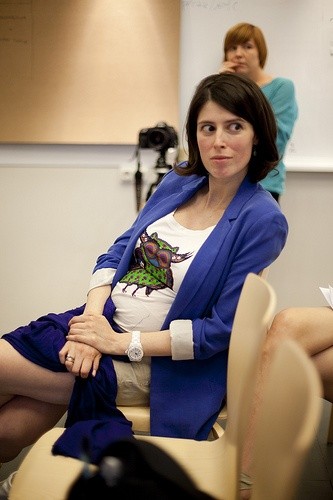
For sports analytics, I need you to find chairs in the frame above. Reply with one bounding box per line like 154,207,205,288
245,335,324,500
97,267,265,440
7,272,276,500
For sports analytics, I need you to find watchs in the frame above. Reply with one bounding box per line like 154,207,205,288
124,330,144,364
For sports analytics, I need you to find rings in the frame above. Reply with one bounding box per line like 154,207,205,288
65,355,74,363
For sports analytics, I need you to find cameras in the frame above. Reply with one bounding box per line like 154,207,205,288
139,125,179,150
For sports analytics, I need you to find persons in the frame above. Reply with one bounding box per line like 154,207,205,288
220,22,299,208
265,304,333,406
0,69,290,466
